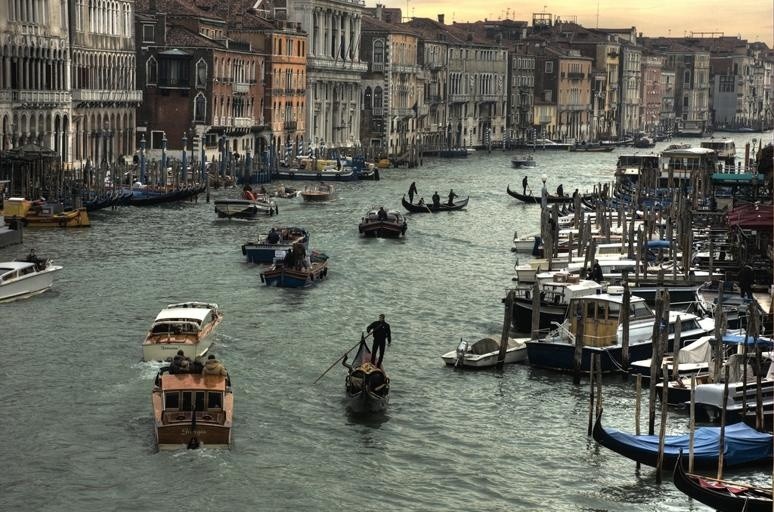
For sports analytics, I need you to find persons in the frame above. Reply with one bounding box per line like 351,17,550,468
366,314,391,368
557,184,563,197
417,198,425,206
26,249,41,269
202,355,227,375
283,242,312,274
572,189,581,198
267,228,278,244
432,191,440,207
368,205,386,220
408,182,417,204
168,350,203,374
336,156,341,170
522,176,527,196
447,189,458,206
587,259,603,282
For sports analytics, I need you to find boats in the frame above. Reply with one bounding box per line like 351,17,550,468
343,332,390,415
358,205,408,239
0,251,63,307
141,298,222,361
442,334,532,370
444,148,477,156
257,241,332,291
401,191,472,217
149,363,234,452
243,225,308,265
